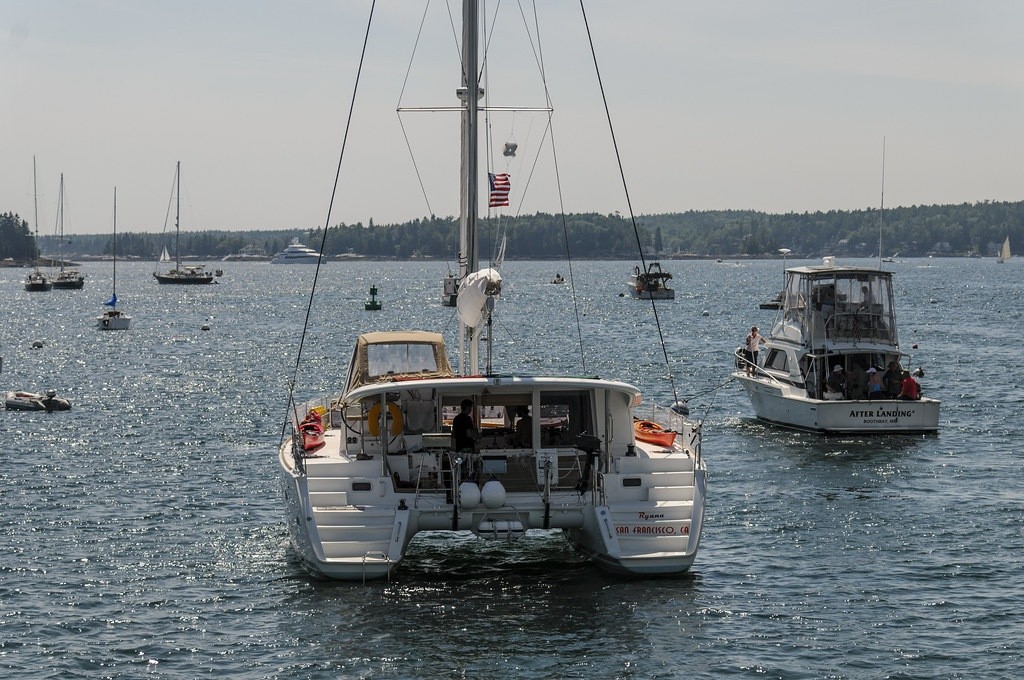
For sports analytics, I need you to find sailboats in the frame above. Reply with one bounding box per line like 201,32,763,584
996,236,1011,265
24,154,53,291
275,0,708,583
49,173,84,289
97,186,131,330
153,161,213,283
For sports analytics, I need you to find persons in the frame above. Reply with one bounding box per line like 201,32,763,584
860,286,876,311
514,408,532,448
882,361,901,399
746,327,766,376
897,371,917,400
827,359,865,400
451,398,483,480
866,367,882,400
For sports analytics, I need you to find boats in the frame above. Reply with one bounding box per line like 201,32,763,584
270,236,325,264
731,136,941,435
716,259,721,262
760,288,787,309
4,392,72,412
882,256,894,262
627,258,675,299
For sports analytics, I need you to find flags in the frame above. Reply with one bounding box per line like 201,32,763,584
488,173,511,207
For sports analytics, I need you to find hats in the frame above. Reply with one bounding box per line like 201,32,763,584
887,361,897,368
866,368,877,373
833,365,843,372
